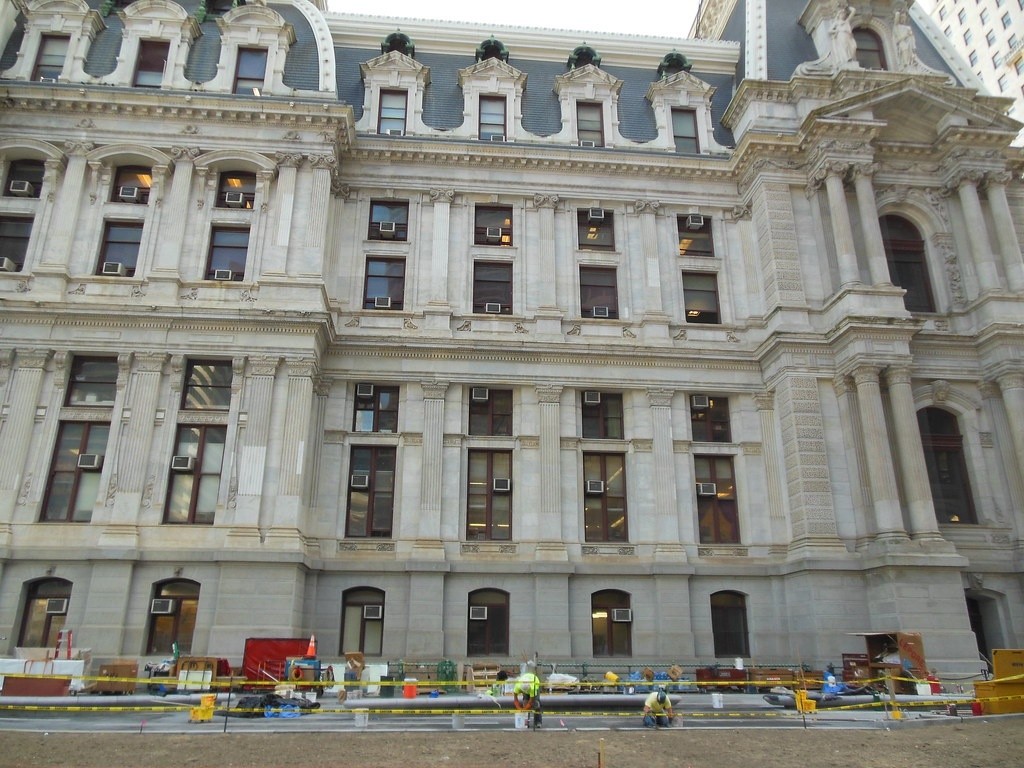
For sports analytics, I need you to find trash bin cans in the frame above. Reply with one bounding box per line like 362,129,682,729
404,679,417,698
380,676,394,697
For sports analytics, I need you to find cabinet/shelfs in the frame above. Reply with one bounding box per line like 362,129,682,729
97,663,139,695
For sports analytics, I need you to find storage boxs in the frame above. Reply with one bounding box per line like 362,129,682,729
973,649,1024,713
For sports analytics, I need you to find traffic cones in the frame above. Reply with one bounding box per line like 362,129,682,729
304,633,318,659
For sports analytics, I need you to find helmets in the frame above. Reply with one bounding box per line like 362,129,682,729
657,691,666,703
521,680,529,692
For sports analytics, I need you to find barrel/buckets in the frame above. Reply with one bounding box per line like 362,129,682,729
403,678,417,698
306,692,317,703
734,658,743,669
514,712,529,729
354,713,369,727
452,715,464,729
604,671,619,681
712,693,723,709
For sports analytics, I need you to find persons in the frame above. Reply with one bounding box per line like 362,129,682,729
512,673,542,728
822,664,848,693
345,657,364,690
643,688,673,728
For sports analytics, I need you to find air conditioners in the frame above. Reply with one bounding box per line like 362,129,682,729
486,227,502,242
692,394,710,409
351,384,374,488
697,480,717,497
40,76,57,83
585,479,605,495
584,391,601,404
103,261,127,275
362,606,381,620
608,607,633,621
485,303,501,313
593,305,609,318
467,606,489,622
170,453,195,472
148,598,176,614
47,598,70,614
493,478,510,491
375,222,395,309
118,186,142,202
474,386,488,402
78,453,100,470
215,270,234,280
588,209,604,222
0,255,17,272
9,180,34,196
686,215,703,229
224,191,245,206
387,128,594,149
